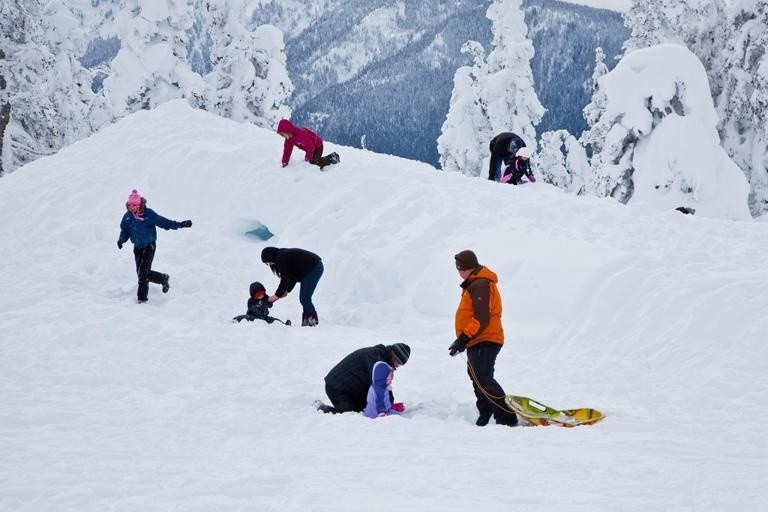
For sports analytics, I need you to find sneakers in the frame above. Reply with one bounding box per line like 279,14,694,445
160,274,170,293
476,412,519,427
329,152,340,164
312,398,323,411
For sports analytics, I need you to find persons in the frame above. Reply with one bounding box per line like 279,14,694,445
500,147,536,185
246,281,292,325
487,132,527,183
314,341,410,414
260,246,324,326
446,249,518,426
363,361,402,418
277,118,340,171
116,189,192,304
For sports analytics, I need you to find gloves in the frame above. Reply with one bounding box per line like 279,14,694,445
518,174,537,184
117,240,126,250
179,219,192,228
447,333,473,358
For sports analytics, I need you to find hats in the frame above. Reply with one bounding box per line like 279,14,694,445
453,249,481,272
506,138,524,153
389,342,410,366
515,147,530,159
126,193,142,206
